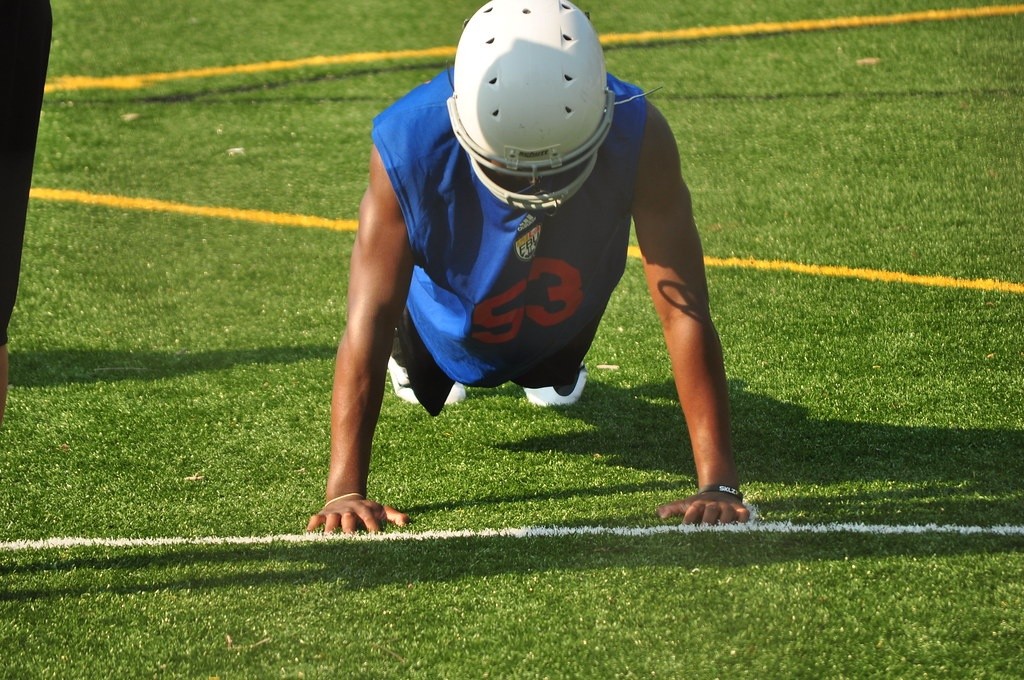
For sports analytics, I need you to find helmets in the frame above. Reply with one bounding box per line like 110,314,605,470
447,0,615,211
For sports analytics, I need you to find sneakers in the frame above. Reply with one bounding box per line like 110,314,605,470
388,355,465,406
523,362,587,408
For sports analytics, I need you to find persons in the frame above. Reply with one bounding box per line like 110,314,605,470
0,0,54,439
302,0,751,533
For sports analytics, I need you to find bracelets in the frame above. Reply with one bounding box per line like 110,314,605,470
696,485,744,502
325,493,365,507
